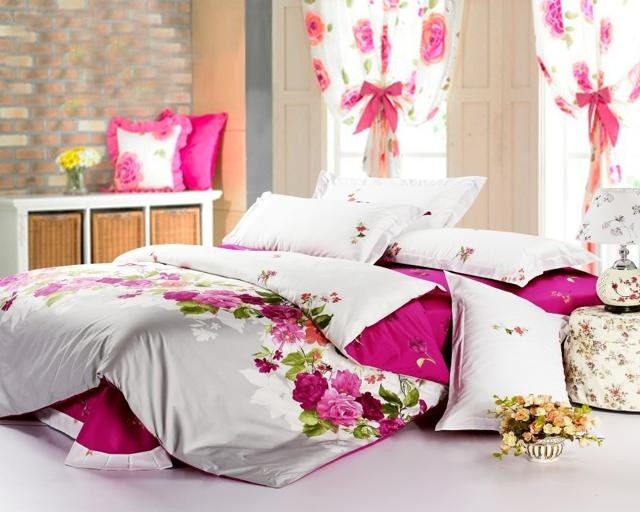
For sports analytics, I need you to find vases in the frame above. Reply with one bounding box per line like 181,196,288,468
61,164,88,197
519,437,567,463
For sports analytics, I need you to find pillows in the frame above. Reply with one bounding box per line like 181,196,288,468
431,267,577,436
308,171,487,232
98,115,191,193
226,189,421,266
386,225,603,287
159,107,229,191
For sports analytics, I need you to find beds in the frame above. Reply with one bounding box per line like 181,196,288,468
2,172,605,491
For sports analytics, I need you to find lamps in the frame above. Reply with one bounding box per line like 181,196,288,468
572,188,639,313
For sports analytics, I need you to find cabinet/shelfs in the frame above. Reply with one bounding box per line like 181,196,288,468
1,190,223,279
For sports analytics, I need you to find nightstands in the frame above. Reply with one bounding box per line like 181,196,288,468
565,304,640,417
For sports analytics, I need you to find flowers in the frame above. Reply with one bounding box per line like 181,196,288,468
57,146,99,190
481,390,604,459
